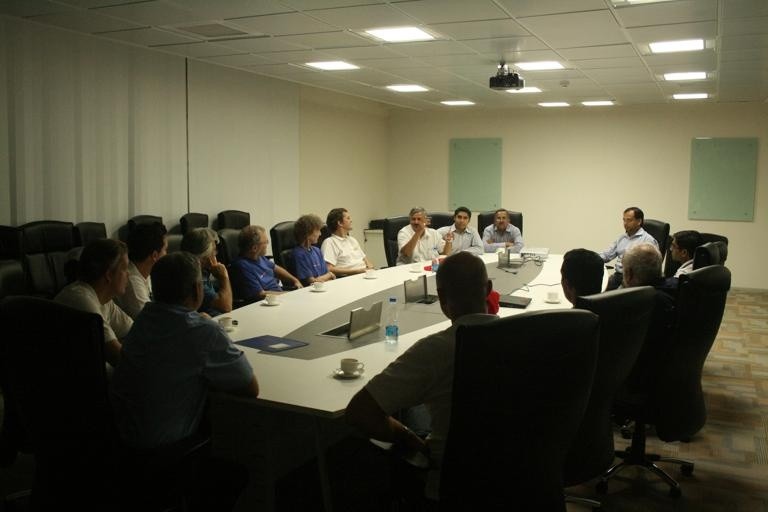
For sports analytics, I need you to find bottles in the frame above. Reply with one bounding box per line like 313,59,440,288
231,320,240,339
614,257,623,273
383,297,402,349
431,247,439,272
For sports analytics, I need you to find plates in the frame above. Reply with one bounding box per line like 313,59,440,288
308,287,326,292
408,269,424,273
331,366,366,380
362,274,377,279
215,327,235,333
260,301,282,306
543,299,562,303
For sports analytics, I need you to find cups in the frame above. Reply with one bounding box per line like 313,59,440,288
365,269,375,275
495,247,505,255
265,294,280,305
217,316,233,329
545,292,558,301
340,357,364,374
409,261,422,270
310,282,325,289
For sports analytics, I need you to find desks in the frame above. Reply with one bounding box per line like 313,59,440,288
211,249,614,512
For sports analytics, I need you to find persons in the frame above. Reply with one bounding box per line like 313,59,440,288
51,225,258,512
396,206,523,266
345,253,503,498
231,209,375,311
559,206,698,440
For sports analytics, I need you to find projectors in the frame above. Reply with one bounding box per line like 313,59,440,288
519,246,549,261
489,73,525,90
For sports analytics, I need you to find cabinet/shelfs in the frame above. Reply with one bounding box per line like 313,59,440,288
359,229,390,271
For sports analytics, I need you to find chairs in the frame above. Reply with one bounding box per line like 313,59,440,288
0,297,264,512
382,209,524,266
382,308,602,512
614,214,731,278
594,265,731,498
555,286,656,512
0,211,330,311
